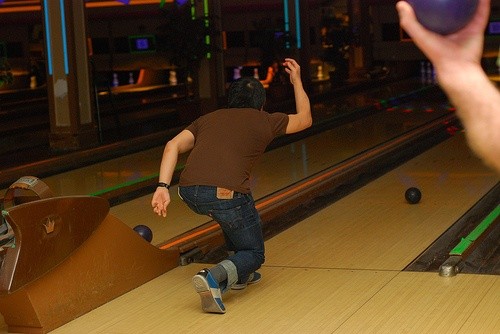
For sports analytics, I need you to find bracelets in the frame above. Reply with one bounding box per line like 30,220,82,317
156,182,171,189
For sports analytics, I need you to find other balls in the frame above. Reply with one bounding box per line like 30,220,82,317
405,188,421,203
133,225,152,242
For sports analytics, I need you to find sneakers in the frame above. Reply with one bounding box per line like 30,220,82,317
192,267,226,313
230,271,262,289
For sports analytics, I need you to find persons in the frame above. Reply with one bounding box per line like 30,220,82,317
153,59,312,313
398,0,500,176
